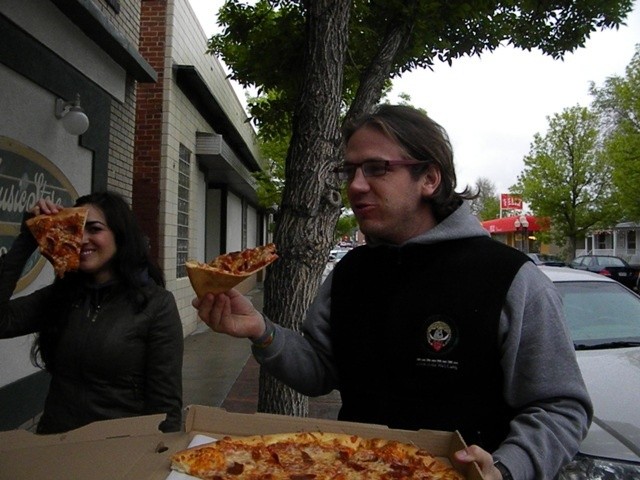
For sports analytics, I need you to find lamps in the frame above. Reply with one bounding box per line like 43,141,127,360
54,91,89,136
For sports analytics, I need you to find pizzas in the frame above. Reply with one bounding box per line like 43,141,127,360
171,433,462,476
185,243,280,298
26,205,91,279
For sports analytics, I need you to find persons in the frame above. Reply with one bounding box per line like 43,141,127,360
0,189,183,436
192,105,593,480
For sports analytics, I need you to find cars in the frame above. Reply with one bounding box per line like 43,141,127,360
535,264,639,480
331,251,347,267
525,253,564,266
568,255,638,289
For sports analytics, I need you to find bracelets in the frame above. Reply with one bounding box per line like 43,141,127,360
491,456,514,480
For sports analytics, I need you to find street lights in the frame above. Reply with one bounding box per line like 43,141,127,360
514,215,529,253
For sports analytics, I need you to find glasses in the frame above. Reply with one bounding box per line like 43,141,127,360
333,160,431,178
